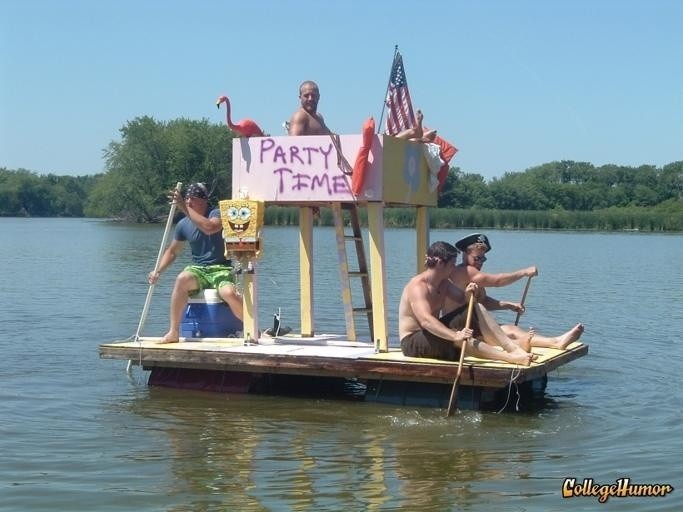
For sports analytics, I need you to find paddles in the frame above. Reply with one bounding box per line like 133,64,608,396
444,292,476,416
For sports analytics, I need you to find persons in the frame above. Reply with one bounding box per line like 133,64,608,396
398,240,540,367
146,181,261,345
287,79,437,141
441,234,585,351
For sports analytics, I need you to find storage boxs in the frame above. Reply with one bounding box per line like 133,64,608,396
176,288,244,340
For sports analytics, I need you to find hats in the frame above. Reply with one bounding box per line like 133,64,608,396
456,234,491,253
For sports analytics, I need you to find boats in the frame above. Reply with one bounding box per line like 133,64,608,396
98,329,587,413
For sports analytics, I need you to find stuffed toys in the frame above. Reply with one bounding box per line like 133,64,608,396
215,95,268,140
216,198,263,275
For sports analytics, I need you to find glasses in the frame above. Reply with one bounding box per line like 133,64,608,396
470,254,487,262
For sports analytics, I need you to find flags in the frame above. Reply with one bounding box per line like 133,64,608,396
375,44,417,137
